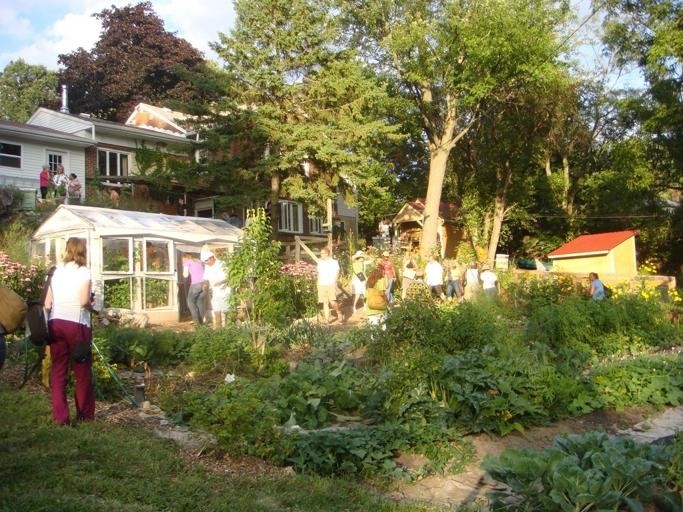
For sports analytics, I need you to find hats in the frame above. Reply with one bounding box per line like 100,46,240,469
199,246,214,262
351,250,366,260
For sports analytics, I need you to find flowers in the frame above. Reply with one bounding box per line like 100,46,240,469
0,249,56,305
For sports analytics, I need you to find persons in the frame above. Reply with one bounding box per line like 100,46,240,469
67,173,82,205
351,250,371,313
108,187,120,208
40,164,52,199
200,251,230,332
43,237,100,425
181,252,205,328
314,248,344,323
376,251,501,304
52,165,67,197
589,272,605,301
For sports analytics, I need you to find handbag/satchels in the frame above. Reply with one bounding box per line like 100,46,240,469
24,265,57,347
603,283,612,298
